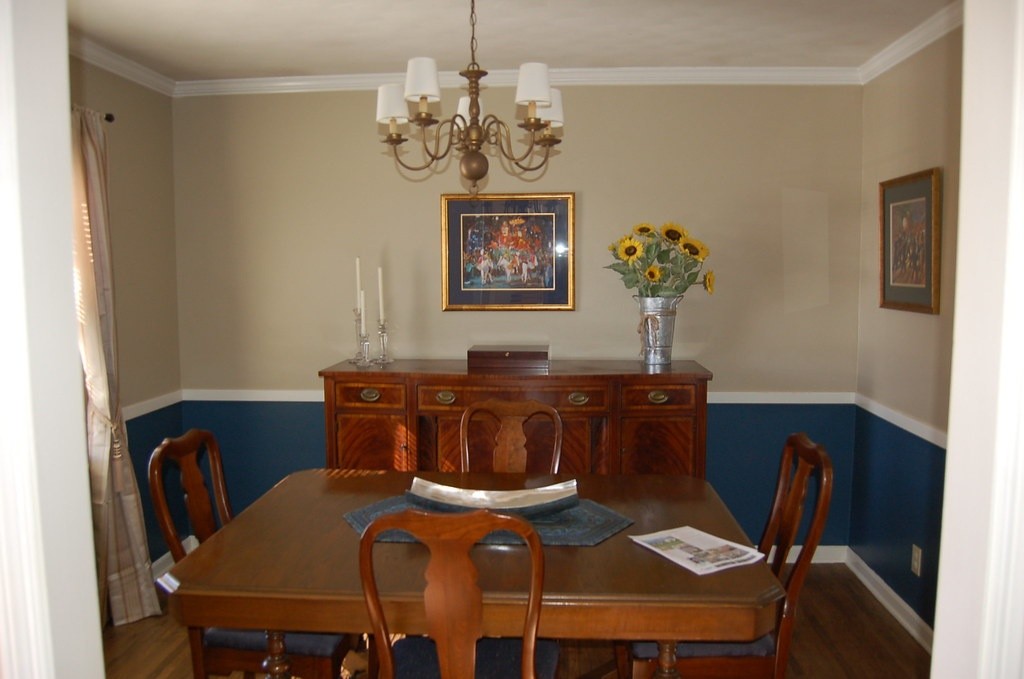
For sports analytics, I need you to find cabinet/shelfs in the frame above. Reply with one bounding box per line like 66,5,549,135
318,357,712,475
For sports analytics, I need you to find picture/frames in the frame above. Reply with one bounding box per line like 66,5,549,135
439,190,578,316
876,165,940,314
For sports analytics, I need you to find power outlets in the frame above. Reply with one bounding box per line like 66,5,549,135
911,544,922,577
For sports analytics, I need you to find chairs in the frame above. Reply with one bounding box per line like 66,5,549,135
613,430,834,677
147,430,349,679
359,506,560,679
461,401,563,474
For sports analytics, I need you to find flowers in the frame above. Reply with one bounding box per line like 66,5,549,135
606,224,717,297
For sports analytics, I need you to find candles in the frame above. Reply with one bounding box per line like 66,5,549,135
379,265,384,323
360,290,367,335
356,256,362,316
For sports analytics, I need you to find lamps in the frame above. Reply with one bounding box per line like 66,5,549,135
377,1,564,184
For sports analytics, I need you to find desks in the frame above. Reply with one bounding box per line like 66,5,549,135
154,467,786,678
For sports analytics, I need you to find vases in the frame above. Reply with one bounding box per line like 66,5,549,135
638,288,679,368
